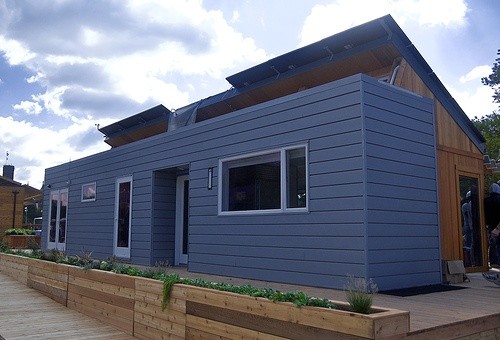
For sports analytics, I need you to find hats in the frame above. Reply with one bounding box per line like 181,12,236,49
466,191,471,198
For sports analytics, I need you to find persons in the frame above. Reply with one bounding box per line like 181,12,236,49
459,179,500,268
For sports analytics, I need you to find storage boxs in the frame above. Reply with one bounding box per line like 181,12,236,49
442,259,466,284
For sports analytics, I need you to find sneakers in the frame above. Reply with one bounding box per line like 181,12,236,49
481,271,500,286
